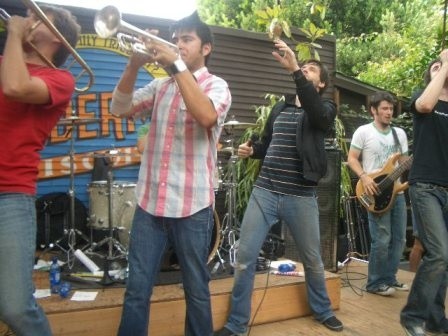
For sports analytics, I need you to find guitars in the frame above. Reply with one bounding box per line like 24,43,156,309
354,152,412,213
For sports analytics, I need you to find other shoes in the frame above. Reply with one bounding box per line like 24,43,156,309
392,282,409,291
428,318,448,333
375,284,396,295
323,316,343,330
214,327,237,336
402,323,427,336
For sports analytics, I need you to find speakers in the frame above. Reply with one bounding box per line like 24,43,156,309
281,148,342,273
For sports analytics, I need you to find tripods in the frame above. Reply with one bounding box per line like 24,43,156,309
218,125,242,274
35,121,128,277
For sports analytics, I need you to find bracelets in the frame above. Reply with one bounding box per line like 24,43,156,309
358,172,367,179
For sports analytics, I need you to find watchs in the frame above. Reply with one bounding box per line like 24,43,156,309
164,58,187,76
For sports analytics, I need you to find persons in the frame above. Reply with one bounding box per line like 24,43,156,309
109,17,232,336
400,49,448,336
348,92,410,295
215,36,343,336
0,5,81,336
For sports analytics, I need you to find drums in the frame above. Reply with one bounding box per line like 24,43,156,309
117,193,222,271
88,179,138,230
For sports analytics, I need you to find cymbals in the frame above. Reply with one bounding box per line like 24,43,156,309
221,116,260,132
79,148,143,160
56,109,105,125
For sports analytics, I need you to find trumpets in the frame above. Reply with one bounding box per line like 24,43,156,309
95,4,180,67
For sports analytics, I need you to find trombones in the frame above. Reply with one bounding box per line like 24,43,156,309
0,0,95,93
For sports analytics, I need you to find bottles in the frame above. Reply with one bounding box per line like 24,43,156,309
59,280,72,296
279,263,296,272
50,257,61,293
